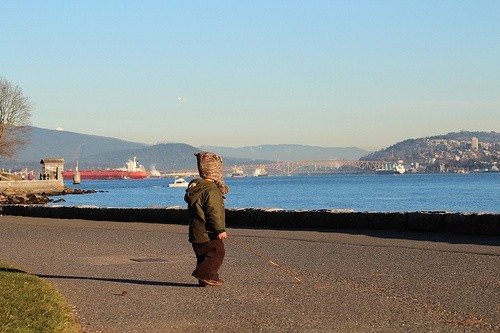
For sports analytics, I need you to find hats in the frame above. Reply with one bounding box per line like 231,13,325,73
194,151,228,194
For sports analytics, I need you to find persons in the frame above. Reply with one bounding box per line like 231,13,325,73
184,150,229,287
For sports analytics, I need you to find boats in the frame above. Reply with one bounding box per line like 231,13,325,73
63,156,268,179
168,177,190,187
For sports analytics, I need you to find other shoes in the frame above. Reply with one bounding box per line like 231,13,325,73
192,271,223,286
